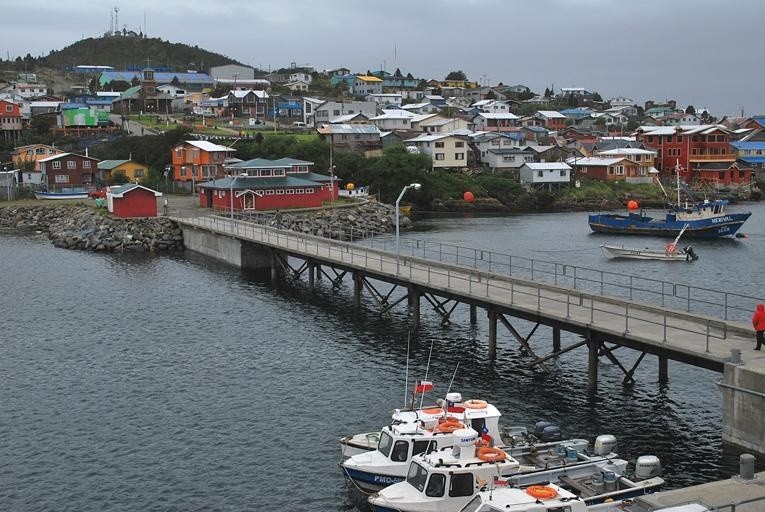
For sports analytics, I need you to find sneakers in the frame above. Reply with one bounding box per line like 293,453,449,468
753,347,761,351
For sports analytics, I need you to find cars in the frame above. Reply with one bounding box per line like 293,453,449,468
292,122,307,128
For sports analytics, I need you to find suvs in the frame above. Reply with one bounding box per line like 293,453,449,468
90,186,121,199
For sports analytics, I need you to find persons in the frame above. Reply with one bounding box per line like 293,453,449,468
752,303,765,350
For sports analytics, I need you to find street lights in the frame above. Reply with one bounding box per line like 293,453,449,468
393,183,422,257
146,105,156,127
3,166,11,201
230,173,248,219
223,137,241,179
190,111,196,133
165,167,171,207
182,166,195,207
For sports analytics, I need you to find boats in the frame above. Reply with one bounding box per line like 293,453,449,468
588,156,754,239
599,223,697,262
338,182,413,216
31,190,90,200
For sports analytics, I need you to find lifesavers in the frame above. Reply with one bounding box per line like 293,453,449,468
439,417,465,433
527,486,557,499
477,447,506,462
465,399,487,409
